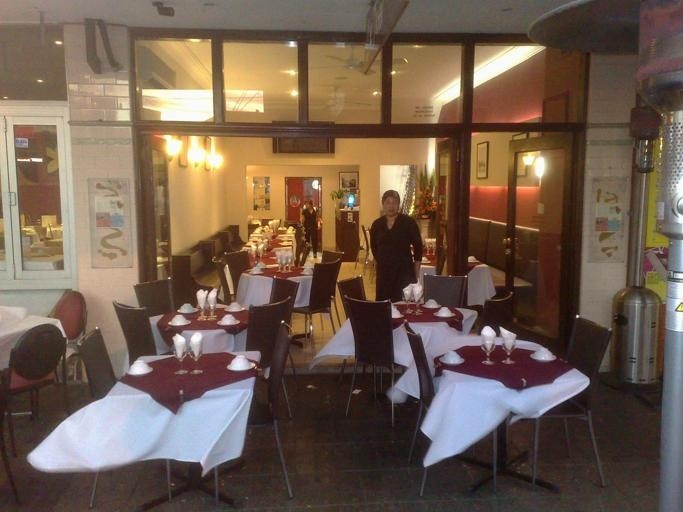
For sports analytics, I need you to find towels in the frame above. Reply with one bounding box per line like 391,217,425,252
190,332,202,357
196,289,209,309
208,288,218,309
480,326,496,352
500,326,517,352
403,283,412,300
413,283,424,302
172,333,186,359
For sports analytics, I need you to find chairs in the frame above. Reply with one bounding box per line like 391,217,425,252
85,327,174,508
343,295,407,427
403,320,500,498
112,300,175,368
245,296,295,419
423,274,468,310
481,291,515,336
133,279,174,320
35,292,94,419
502,315,611,489
1,370,21,512
209,320,296,499
211,256,237,305
301,249,345,331
293,258,342,354
267,276,300,382
225,249,250,294
8,323,72,457
336,276,383,400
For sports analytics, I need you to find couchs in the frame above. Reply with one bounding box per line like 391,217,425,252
199,235,238,269
490,221,538,291
216,224,246,253
468,217,490,264
173,246,223,307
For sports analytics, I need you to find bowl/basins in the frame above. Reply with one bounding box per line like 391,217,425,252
425,299,438,307
228,302,241,309
533,348,553,360
180,303,194,311
257,262,266,267
129,360,150,373
222,314,235,323
443,351,462,363
231,355,250,368
303,268,313,273
253,267,261,272
171,315,186,323
437,307,453,315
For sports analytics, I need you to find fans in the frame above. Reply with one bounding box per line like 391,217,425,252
308,45,409,75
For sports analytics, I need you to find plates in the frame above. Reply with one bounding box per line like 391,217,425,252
225,362,256,372
248,271,264,274
300,265,314,269
421,305,442,308
177,308,200,314
216,320,241,326
432,312,456,318
304,262,312,267
223,307,245,312
438,357,465,367
300,272,313,275
529,354,557,362
167,319,192,326
125,367,154,377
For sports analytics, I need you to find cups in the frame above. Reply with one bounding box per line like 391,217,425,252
502,339,517,364
171,343,189,375
275,255,283,273
413,290,424,316
401,291,413,314
280,256,289,274
479,338,496,366
414,297,424,313
188,342,203,375
196,303,207,321
207,302,217,321
289,256,295,273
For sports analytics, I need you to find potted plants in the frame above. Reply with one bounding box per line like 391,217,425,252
331,189,345,208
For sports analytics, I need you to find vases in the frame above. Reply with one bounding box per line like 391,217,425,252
418,216,430,244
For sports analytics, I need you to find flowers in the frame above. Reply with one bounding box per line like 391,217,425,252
411,164,437,221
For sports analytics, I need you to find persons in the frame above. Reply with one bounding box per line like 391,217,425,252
371,190,423,303
300,201,323,258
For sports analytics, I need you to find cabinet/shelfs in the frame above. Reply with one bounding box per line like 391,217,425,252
336,208,360,263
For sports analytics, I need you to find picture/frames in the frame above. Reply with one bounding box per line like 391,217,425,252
475,140,490,180
338,171,360,191
512,131,529,179
179,136,189,168
205,137,212,170
